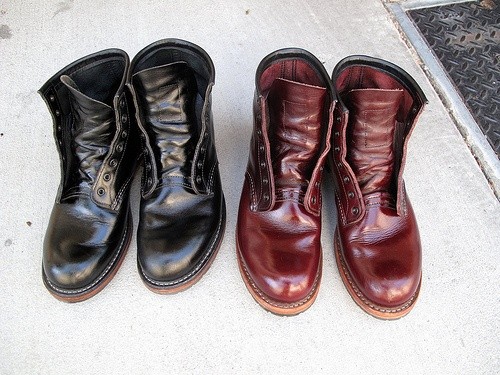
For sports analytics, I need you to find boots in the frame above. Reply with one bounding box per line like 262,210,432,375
234,47,338,317
37,48,142,303
331,54,422,319
124,38,226,296
